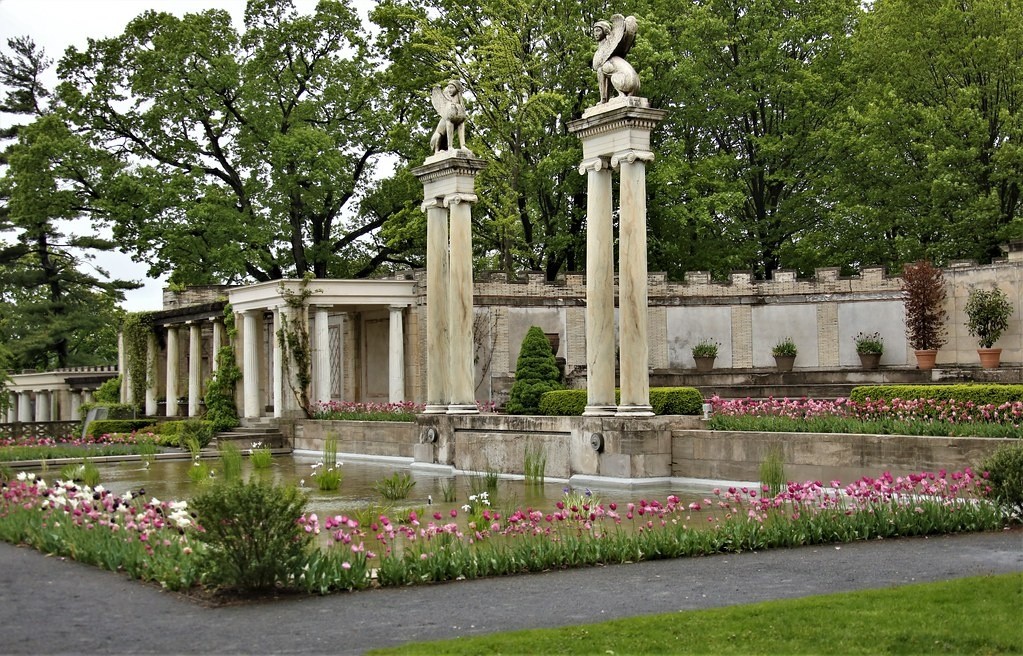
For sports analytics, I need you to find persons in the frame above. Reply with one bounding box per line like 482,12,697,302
430,79,466,154
593,21,642,105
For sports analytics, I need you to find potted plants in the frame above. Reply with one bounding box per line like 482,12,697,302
964,283,1014,368
690,338,722,372
769,335,798,372
896,260,950,368
853,332,885,370
